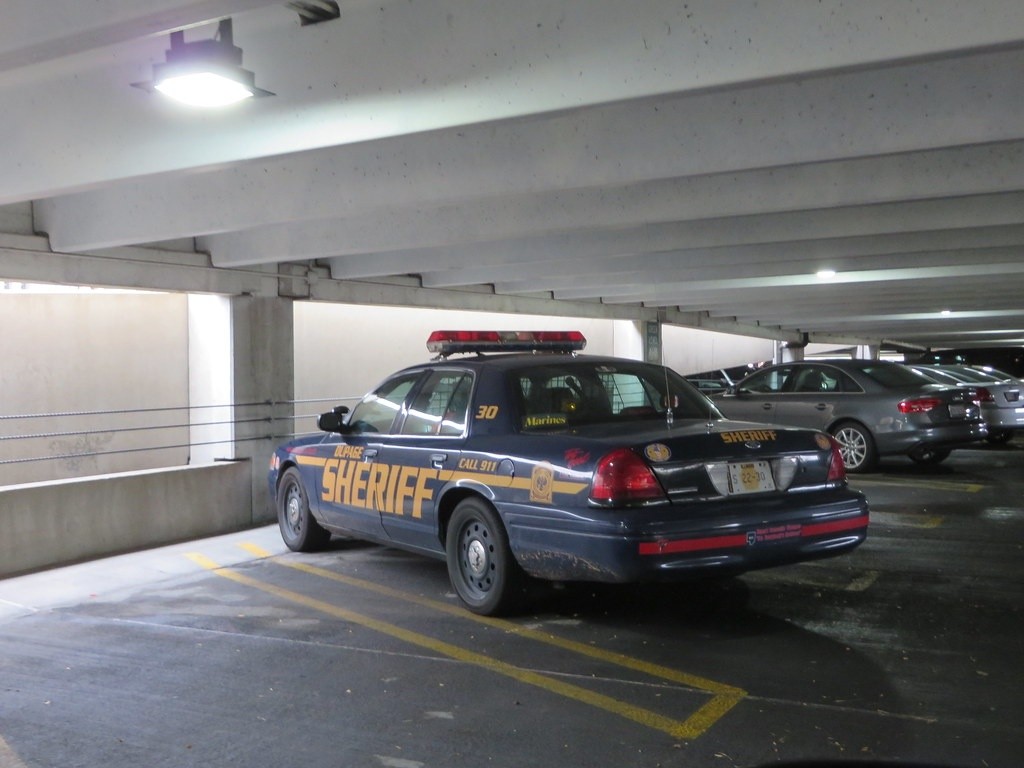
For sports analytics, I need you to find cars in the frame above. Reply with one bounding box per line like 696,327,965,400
686,359,1024,473
267,331,870,615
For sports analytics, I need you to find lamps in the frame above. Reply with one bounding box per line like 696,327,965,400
128,17,276,109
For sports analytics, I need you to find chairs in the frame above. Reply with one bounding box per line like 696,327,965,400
794,371,821,392
539,386,575,412
